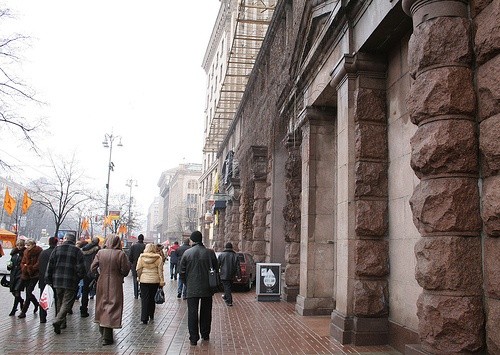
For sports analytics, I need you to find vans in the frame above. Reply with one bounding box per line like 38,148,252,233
57,229,77,243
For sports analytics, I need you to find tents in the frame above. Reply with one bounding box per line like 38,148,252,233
0,228,17,248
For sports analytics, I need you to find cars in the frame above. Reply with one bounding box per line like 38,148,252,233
215,251,256,291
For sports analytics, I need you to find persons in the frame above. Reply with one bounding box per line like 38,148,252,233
90,233,131,345
7,233,101,335
119,234,192,299
218,242,242,306
179,231,219,346
135,242,165,325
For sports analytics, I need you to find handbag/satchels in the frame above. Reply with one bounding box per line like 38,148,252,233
0,274,11,288
208,266,221,290
154,288,165,305
39,284,55,314
88,278,97,294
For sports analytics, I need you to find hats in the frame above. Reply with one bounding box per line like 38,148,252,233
189,231,203,242
137,234,144,241
48,236,59,246
183,238,190,245
225,242,233,248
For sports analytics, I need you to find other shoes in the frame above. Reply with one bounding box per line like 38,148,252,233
222,295,233,306
101,338,114,346
170,273,178,280
177,292,182,298
39,314,47,324
189,337,198,346
52,319,66,334
200,330,211,340
134,293,144,300
80,309,90,317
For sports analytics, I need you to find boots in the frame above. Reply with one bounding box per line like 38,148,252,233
10,296,25,316
30,294,40,314
18,303,29,318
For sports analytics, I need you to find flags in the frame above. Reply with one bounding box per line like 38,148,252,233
20,190,32,214
81,216,88,231
101,214,128,233
4,186,17,216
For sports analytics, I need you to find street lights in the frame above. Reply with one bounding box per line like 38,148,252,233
125,179,138,241
103,133,122,241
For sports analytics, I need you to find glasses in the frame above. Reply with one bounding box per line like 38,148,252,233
60,241,63,243
